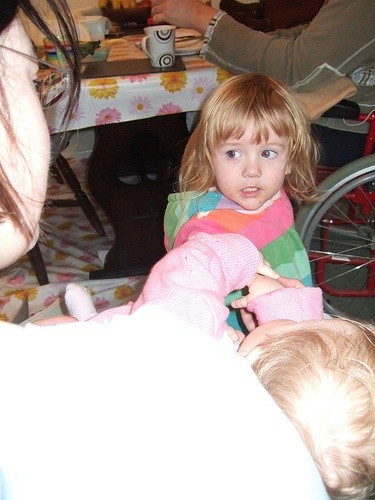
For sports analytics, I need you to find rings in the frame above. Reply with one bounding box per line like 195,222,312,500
155,7,160,14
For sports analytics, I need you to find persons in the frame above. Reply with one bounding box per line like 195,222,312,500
0,1,331,500
150,0,375,170
164,72,315,333
89,234,374,500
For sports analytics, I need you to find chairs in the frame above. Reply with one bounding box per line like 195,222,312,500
0,13,106,286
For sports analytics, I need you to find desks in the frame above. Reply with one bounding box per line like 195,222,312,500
37,27,236,280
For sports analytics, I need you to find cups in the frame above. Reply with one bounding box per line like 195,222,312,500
141,25,176,67
43,35,74,72
80,16,111,41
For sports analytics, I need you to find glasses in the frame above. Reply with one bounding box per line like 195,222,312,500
0,44,73,108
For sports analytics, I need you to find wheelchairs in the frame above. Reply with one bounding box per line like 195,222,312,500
295,99,375,327
